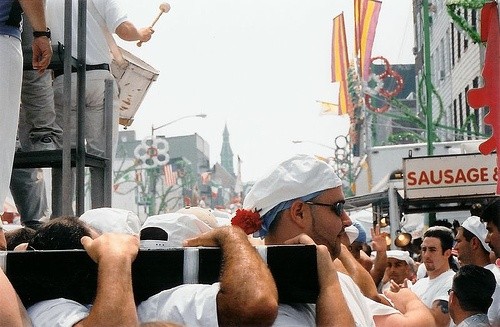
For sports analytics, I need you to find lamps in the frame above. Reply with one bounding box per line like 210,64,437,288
379,213,411,248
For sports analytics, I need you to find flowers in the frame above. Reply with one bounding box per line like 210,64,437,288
231,208,263,234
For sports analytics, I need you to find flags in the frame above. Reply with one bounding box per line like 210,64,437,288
164,163,177,185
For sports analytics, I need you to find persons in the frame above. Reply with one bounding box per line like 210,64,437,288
243,153,500,327
0,0,52,214
9,0,155,226
1,206,279,327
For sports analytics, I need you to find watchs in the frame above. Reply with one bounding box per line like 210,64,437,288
33,27,51,38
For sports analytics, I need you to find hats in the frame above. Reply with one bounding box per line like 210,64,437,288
138,212,215,250
384,250,414,265
350,220,366,242
176,206,216,229
78,208,141,246
461,215,493,253
242,154,342,222
204,208,233,223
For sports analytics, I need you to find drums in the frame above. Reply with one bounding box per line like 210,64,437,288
109,45,161,130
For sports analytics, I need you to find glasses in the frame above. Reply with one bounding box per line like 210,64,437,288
303,200,348,216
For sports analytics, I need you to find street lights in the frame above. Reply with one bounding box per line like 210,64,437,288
293,140,339,177
149,113,207,215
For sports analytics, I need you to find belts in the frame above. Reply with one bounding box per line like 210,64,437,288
71,64,109,73
